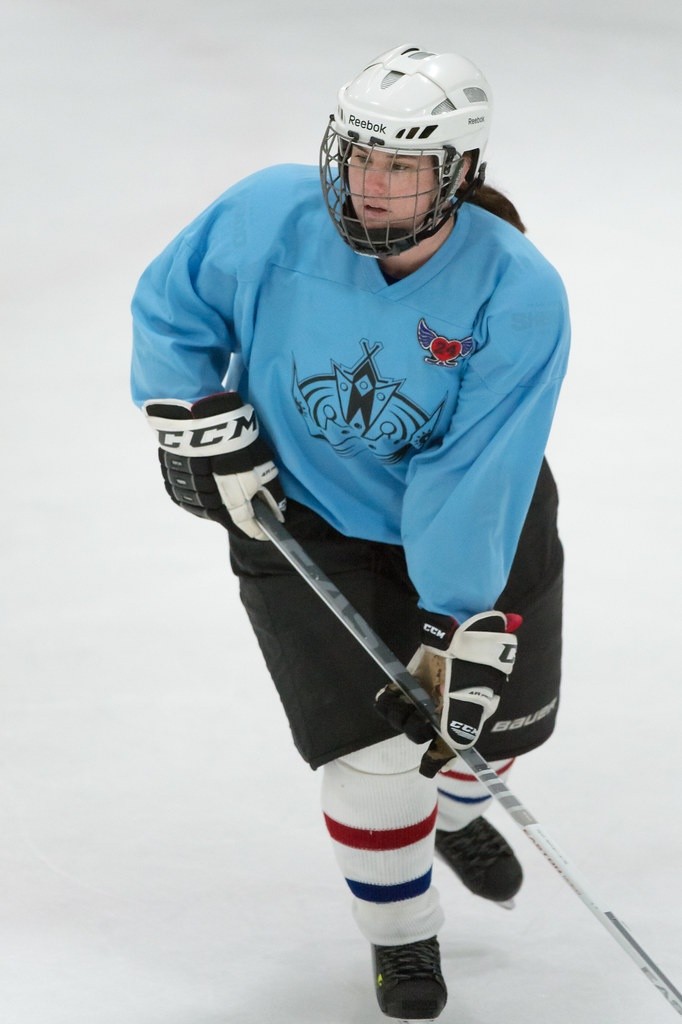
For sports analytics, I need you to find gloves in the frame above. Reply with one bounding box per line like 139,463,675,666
372,606,518,778
141,386,290,541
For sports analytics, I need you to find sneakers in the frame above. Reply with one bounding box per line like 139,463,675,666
432,817,524,910
368,938,448,1024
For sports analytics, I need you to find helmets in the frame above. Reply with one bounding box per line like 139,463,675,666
318,45,495,257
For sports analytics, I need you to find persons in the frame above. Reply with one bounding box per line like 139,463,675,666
132,41,570,1023
